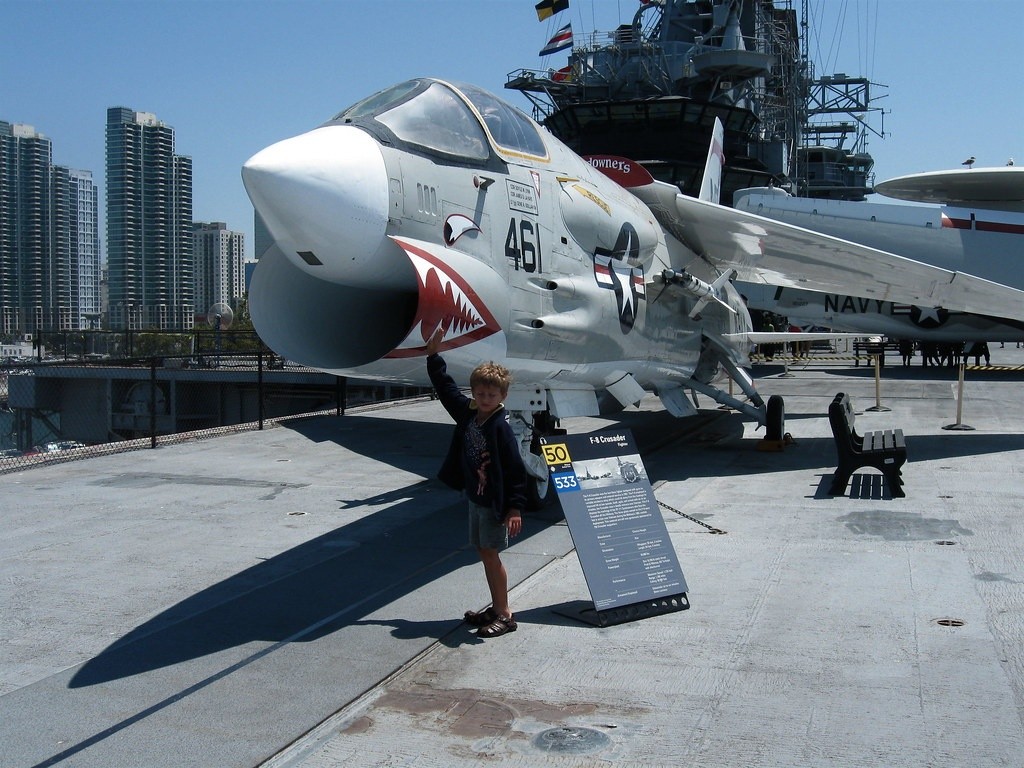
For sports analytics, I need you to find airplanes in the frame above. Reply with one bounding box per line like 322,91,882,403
242,78,1024,512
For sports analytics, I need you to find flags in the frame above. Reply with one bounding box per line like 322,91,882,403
535,0,569,22
539,22,574,56
552,65,579,82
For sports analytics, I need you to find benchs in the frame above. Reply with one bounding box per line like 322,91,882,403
829,393,906,499
853,336,914,367
916,340,984,366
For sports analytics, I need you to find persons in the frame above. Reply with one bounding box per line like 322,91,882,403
1016,342,1024,348
938,345,953,367
963,341,991,367
954,345,962,364
749,344,757,362
426,326,527,638
788,326,802,358
928,340,940,367
899,340,912,367
1000,341,1004,348
760,317,775,361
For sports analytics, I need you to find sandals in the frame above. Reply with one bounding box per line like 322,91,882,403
465,607,498,626
478,613,518,638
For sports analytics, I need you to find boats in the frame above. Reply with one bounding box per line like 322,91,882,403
504,0,893,208
